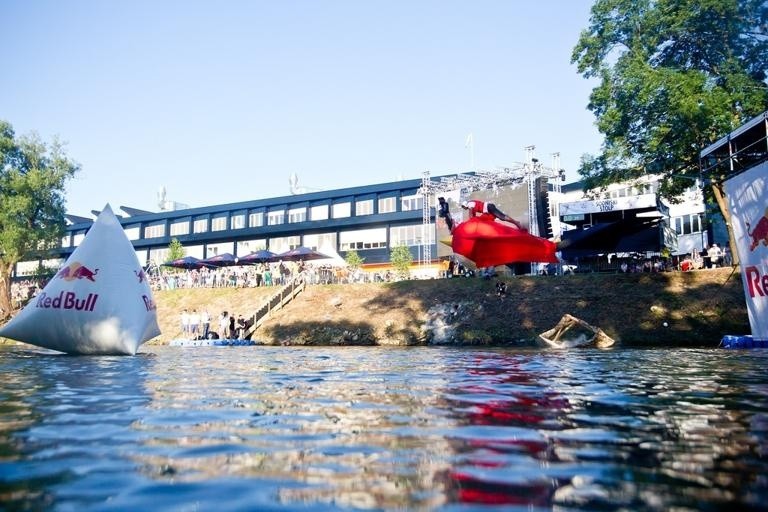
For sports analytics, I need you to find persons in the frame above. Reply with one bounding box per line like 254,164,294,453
495,279,508,302
448,302,460,322
461,199,530,231
8,273,43,306
436,196,457,231
149,259,415,293
537,241,736,275
178,305,248,344
